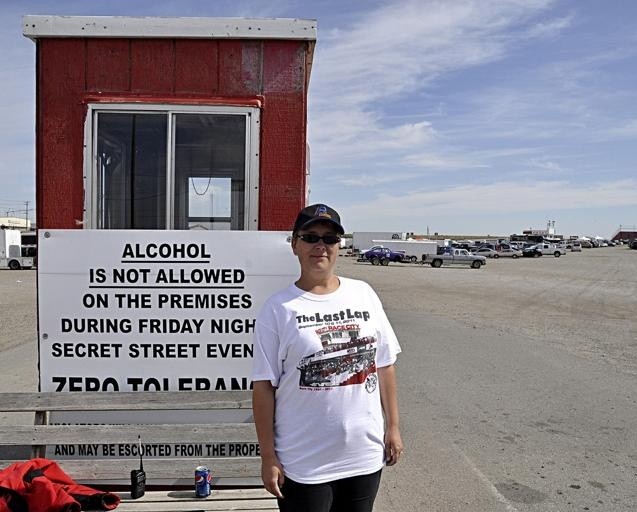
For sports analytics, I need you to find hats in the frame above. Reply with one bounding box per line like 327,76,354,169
294,204,345,235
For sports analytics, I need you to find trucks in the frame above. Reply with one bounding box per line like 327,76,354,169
352,232,498,262
0,228,37,270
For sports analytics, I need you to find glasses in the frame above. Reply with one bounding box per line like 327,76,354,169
294,231,343,245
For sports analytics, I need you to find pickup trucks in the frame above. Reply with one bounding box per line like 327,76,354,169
422,249,487,269
525,241,574,257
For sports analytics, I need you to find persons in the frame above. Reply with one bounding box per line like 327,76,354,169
250,202,404,511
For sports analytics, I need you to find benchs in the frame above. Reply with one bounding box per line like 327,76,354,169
0,390,280,512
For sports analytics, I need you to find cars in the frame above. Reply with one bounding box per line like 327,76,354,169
571,238,637,252
468,247,524,259
360,245,404,262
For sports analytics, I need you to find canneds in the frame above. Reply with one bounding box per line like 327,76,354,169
195,466,210,498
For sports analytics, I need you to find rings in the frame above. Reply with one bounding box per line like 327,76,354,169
399,450,403,454
